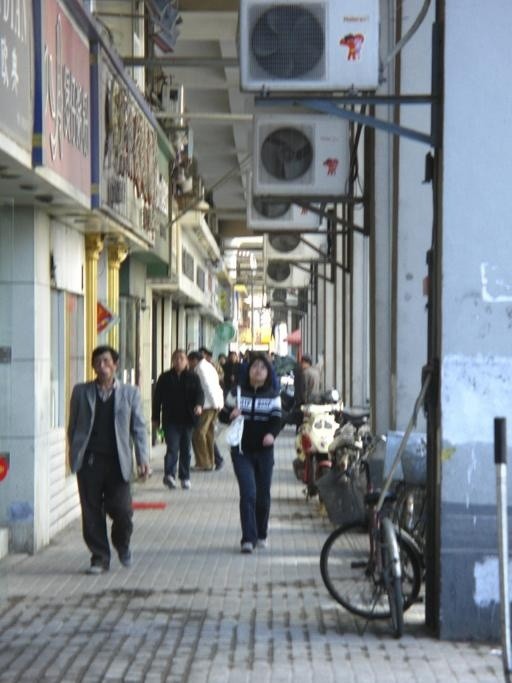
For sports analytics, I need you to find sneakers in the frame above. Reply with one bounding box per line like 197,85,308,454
241,538,267,553
85,551,132,574
162,474,191,490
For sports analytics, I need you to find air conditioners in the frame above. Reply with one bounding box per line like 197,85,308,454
264,233,328,260
247,198,322,230
253,111,350,197
270,290,300,307
264,260,311,289
240,0,379,92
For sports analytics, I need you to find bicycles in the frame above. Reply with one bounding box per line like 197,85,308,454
313,438,428,639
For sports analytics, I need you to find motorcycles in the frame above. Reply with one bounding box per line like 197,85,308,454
292,400,344,498
328,406,373,482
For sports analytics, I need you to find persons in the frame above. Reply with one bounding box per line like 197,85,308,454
65,344,150,576
218,356,285,553
152,343,323,491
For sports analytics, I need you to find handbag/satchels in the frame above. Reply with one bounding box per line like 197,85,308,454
218,386,244,448
